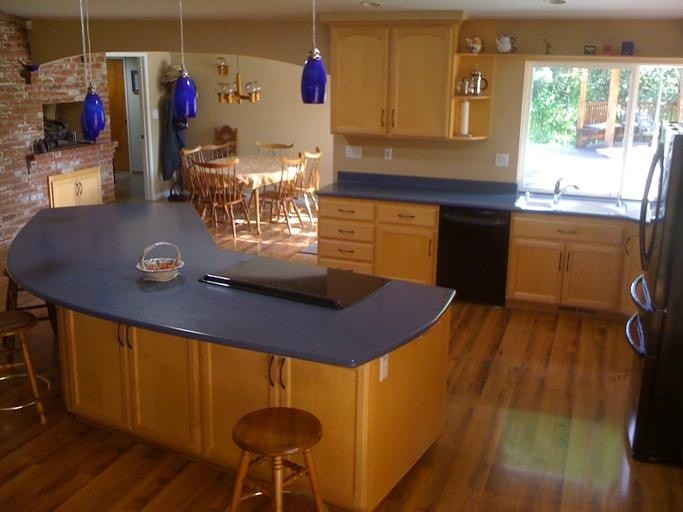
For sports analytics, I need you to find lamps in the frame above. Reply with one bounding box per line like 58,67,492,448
74,0,104,142
213,51,263,106
170,0,197,120
300,1,327,107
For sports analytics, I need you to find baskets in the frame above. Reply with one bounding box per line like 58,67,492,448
135,242,183,281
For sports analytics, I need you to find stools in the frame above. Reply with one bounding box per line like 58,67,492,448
2,267,58,361
228,407,324,510
1,307,52,427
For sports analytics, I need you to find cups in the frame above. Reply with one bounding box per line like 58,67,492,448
471,70,488,96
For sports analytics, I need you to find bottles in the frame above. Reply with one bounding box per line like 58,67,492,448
36,139,49,153
460,78,468,97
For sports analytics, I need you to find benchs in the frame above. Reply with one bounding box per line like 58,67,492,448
583,99,676,150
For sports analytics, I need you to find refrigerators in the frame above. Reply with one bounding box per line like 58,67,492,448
623,118,683,467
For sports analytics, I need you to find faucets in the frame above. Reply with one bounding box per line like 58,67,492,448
553,177,580,205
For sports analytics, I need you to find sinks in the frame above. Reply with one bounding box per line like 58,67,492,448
557,194,628,218
514,195,558,214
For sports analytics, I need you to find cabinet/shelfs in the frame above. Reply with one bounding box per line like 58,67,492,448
447,48,493,141
619,220,662,323
374,199,438,286
47,165,101,210
200,300,451,511
317,196,373,275
323,18,455,139
58,306,199,460
504,212,624,317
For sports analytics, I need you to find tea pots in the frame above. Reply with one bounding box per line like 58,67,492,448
495,33,515,54
466,34,483,55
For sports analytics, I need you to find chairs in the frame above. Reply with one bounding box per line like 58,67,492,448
178,125,322,241
